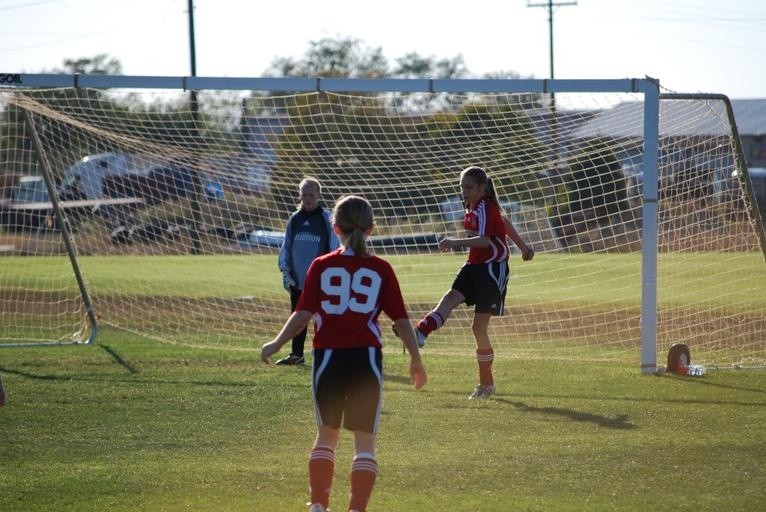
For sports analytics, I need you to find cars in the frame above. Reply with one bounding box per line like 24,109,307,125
14,176,48,202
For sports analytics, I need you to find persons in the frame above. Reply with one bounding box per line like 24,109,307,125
258,197,426,512
276,176,341,366
392,165,534,401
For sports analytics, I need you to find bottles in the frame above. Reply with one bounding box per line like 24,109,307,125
679,365,705,377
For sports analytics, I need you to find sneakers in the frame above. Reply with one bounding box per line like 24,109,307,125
392,321,425,348
275,353,305,366
468,383,497,400
